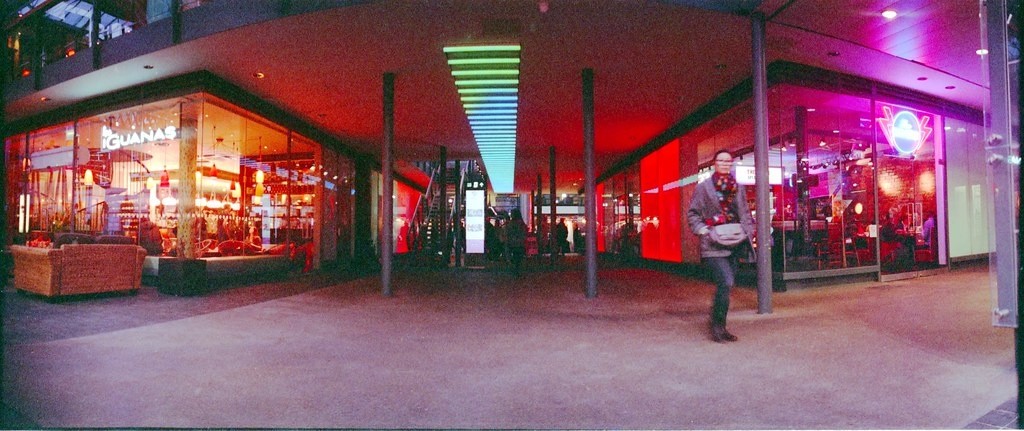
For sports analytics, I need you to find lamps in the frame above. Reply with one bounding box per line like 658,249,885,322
210,127,218,177
230,142,236,191
159,119,170,188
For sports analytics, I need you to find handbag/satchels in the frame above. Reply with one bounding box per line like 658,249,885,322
708,224,748,249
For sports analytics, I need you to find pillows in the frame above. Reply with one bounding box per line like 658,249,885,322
96,235,133,244
52,234,96,250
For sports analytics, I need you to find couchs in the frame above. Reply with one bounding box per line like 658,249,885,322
10,234,148,300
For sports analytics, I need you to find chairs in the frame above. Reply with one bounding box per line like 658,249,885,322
196,239,265,258
813,219,841,270
914,227,936,264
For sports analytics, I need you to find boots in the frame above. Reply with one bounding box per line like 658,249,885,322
711,295,737,344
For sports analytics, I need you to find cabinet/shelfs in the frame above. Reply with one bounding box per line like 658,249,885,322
238,152,314,242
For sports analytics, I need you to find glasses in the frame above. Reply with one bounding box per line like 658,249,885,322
716,159,733,166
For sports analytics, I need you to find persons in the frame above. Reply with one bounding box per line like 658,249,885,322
686,151,757,343
485,208,568,276
137,217,163,255
